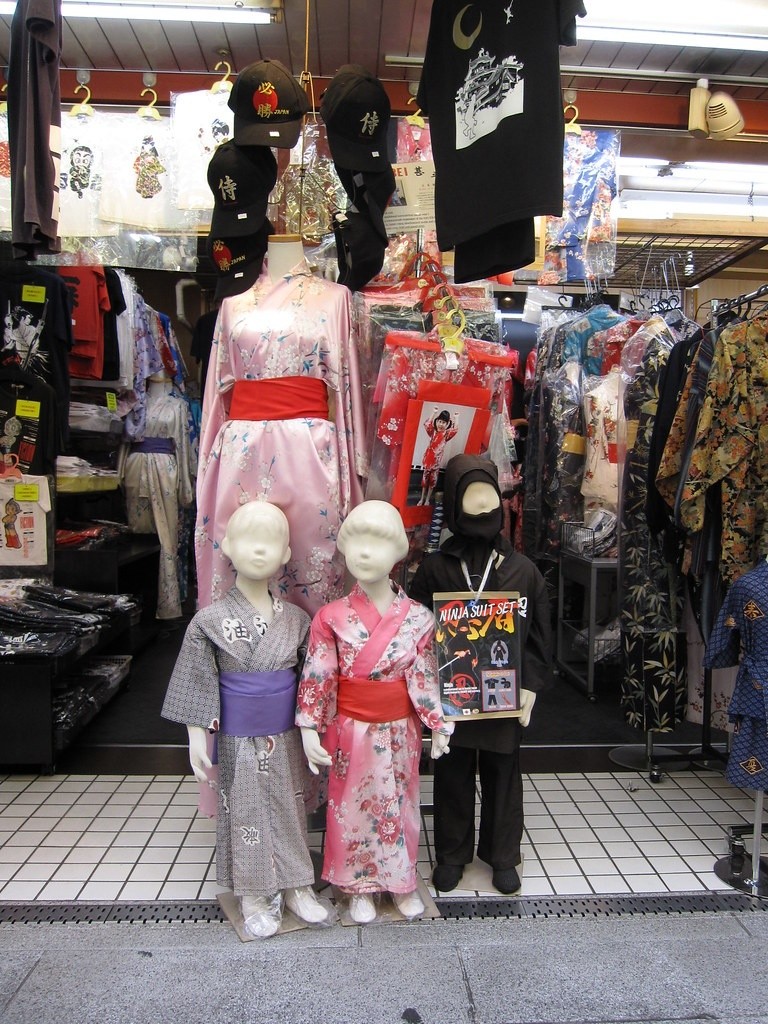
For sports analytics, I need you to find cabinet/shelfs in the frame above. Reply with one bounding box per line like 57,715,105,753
0,386,174,774
556,523,620,702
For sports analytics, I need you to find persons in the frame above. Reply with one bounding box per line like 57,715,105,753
160,501,329,938
114,382,193,619
581,367,621,526
411,455,550,895
706,551,768,795
194,234,363,622
417,407,459,506
291,499,452,923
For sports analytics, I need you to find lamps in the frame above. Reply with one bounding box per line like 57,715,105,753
61,0,284,24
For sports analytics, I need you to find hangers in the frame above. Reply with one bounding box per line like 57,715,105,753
404,97,426,129
627,301,637,315
632,294,652,320
211,61,234,93
558,296,567,313
715,284,768,328
564,105,582,135
578,289,609,311
649,295,679,314
136,88,161,120
69,85,94,116
410,253,466,355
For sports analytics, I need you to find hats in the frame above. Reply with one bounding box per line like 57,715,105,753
319,63,392,172
207,138,278,238
334,163,396,240
206,235,269,304
228,57,310,149
332,211,385,296
